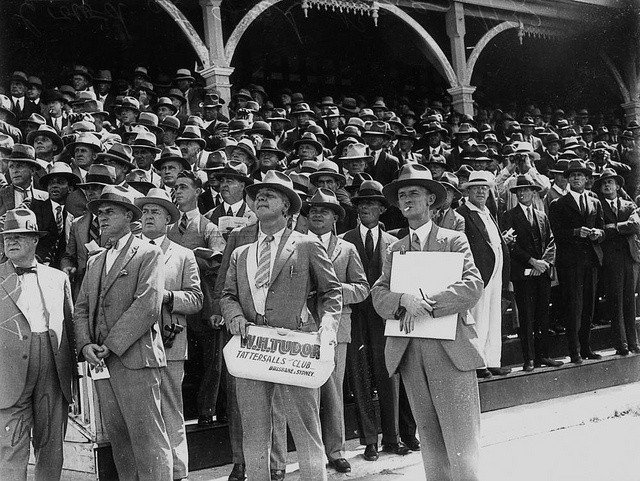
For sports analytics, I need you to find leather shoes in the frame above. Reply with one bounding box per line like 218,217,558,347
402,436,420,451
476,369,492,377
228,464,245,481
523,360,535,373
363,443,379,460
487,367,511,375
329,457,352,473
383,442,409,454
580,351,600,360
628,344,640,354
272,470,285,481
534,358,563,368
616,347,628,355
570,353,583,363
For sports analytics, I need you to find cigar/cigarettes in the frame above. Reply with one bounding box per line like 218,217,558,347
403,207,410,212
96,363,103,367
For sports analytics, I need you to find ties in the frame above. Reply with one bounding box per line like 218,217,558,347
47,164,51,167
56,205,63,235
150,239,154,243
405,152,408,159
365,229,374,261
226,205,235,234
610,202,617,218
15,187,31,201
371,151,376,166
54,117,62,136
180,213,189,235
89,216,99,245
255,236,270,290
410,234,421,251
169,188,178,209
579,195,587,216
432,149,436,154
526,207,532,224
554,157,558,161
15,100,21,114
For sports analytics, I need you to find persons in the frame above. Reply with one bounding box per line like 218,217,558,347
531,161,555,176
345,173,387,233
595,160,616,168
546,159,569,210
432,172,466,233
223,80,399,202
548,159,606,364
60,163,143,303
455,170,517,378
500,176,564,371
134,188,205,481
371,164,487,481
87,64,198,197
584,162,601,200
616,160,639,201
485,186,504,225
593,168,640,356
534,107,640,161
219,170,343,481
300,187,371,473
546,317,567,336
300,160,358,236
422,155,453,182
72,184,173,481
2,144,58,265
450,164,474,210
32,163,75,270
591,304,610,326
198,64,223,214
285,172,310,236
208,219,287,481
399,374,422,451
0,208,75,481
495,142,551,211
399,97,511,173
511,101,534,143
197,329,224,427
0,63,87,214
203,160,259,240
340,180,412,462
165,170,227,252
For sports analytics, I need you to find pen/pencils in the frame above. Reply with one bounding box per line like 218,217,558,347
290,265,294,281
419,288,433,320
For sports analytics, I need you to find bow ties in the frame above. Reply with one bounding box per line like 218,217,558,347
16,267,37,275
105,238,118,247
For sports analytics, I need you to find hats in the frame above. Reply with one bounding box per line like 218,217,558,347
180,115,209,134
174,68,195,83
424,121,449,137
591,168,624,195
207,88,226,104
426,154,449,169
291,103,315,116
81,98,110,117
158,116,181,130
134,188,180,224
45,90,66,104
549,158,570,172
436,171,463,202
382,162,446,210
619,130,638,141
446,109,464,123
0,143,46,171
453,122,478,136
381,111,397,123
266,108,291,123
593,142,613,150
338,97,360,114
355,94,367,102
321,106,344,118
213,159,252,187
298,120,316,131
93,69,112,81
315,96,337,109
228,119,253,135
400,110,418,119
19,113,46,127
365,120,392,139
342,117,366,132
224,138,258,171
462,144,491,162
358,108,379,120
62,121,102,141
86,185,143,223
121,125,150,143
0,131,15,151
350,180,390,208
461,169,494,192
345,172,373,194
481,133,504,148
244,122,274,139
281,88,293,96
293,132,322,155
626,120,640,130
563,136,582,150
509,141,541,161
39,162,82,191
337,132,365,145
59,85,76,97
509,176,541,192
396,127,419,143
559,149,578,160
339,143,372,161
68,92,93,107
289,160,317,178
337,137,360,150
214,137,239,153
0,94,16,121
125,169,155,188
337,126,365,142
202,151,227,170
174,124,207,150
285,93,310,106
474,104,622,137
233,88,253,101
97,142,137,170
67,64,92,88
2,71,31,87
154,73,173,88
384,123,395,136
134,80,156,96
119,97,142,113
130,131,162,153
429,100,448,114
288,171,308,198
245,169,302,215
109,96,125,108
65,132,101,155
153,145,192,171
371,99,388,109
310,160,346,188
131,67,151,82
239,101,261,112
387,117,406,133
301,125,331,144
0,209,49,239
258,100,275,110
463,114,474,122
302,187,347,221
543,133,563,146
199,94,222,109
26,124,65,156
129,111,164,134
153,97,177,115
247,82,268,97
28,76,44,90
563,158,592,180
76,163,115,185
166,89,186,107
256,139,286,161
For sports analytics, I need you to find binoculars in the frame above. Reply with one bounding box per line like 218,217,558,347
509,154,526,165
236,106,253,117
68,113,87,125
161,323,184,348
591,150,605,160
